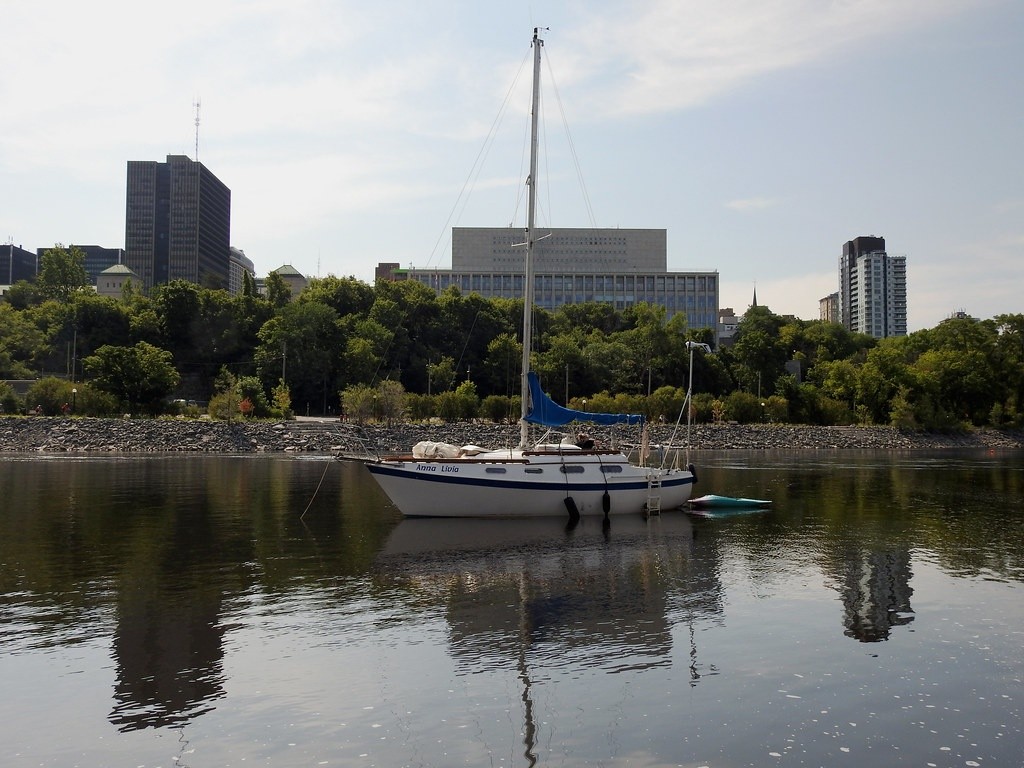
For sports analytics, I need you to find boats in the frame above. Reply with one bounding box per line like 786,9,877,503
687,495,773,508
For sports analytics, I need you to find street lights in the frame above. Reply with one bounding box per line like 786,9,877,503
582,400,587,412
72,388,78,414
761,403,765,424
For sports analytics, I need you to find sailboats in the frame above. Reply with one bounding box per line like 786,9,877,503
362,24,695,519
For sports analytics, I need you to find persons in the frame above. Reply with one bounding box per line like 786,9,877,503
575,434,594,449
659,415,663,422
62,402,71,416
35,405,41,417
